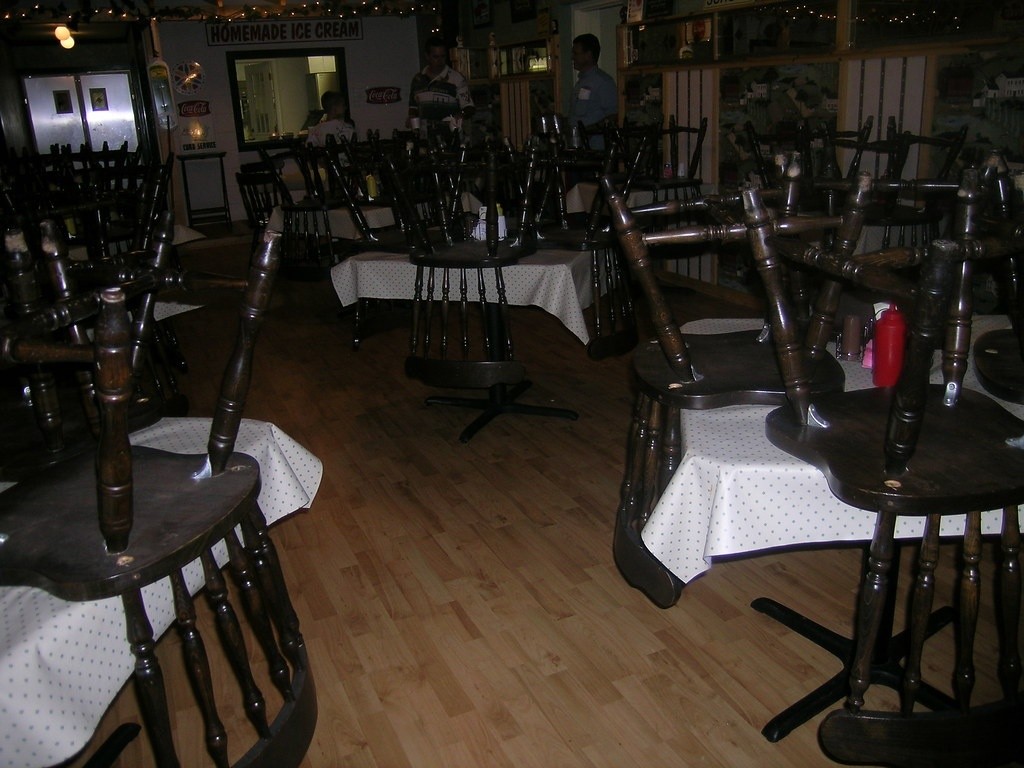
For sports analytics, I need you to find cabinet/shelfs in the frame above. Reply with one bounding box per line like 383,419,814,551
245,57,310,139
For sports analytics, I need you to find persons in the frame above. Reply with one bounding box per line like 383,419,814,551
561,32,616,151
408,38,473,143
305,92,352,172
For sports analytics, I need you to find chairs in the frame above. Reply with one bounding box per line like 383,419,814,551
0,115,1023,768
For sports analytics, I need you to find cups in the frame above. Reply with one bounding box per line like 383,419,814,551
842,314,861,362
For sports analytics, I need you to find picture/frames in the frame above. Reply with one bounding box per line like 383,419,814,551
510,0,537,24
471,0,493,29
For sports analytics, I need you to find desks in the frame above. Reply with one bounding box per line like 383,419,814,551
565,181,700,213
640,320,1024,744
0,417,323,768
329,219,612,445
81,300,206,340
68,224,207,262
266,193,486,330
765,206,946,258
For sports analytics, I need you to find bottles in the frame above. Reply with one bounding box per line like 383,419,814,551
872,303,905,386
6,228,42,313
663,164,672,178
40,222,75,302
367,176,377,198
